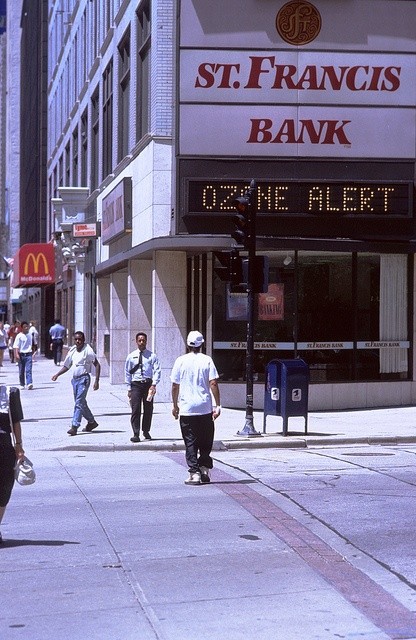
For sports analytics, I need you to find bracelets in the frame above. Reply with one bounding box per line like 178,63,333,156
14,440,23,447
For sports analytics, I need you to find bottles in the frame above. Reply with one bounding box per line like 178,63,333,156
146,390,154,403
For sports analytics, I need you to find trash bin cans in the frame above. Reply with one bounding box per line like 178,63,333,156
264,358,308,436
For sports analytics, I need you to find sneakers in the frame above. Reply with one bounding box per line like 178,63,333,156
67,427,77,434
57,362,60,366
15,362,18,366
82,420,98,431
185,472,201,485
27,384,33,390
199,466,210,482
20,386,24,390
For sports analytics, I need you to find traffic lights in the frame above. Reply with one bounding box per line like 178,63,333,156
228,197,257,251
210,250,240,280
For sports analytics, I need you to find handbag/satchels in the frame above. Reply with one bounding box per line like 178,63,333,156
13,456,36,485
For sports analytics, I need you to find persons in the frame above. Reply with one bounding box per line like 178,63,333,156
170,329,223,485
0,380,27,537
123,332,161,442
12,321,39,390
7,336,15,364
4,321,10,345
51,330,102,436
0,320,8,368
8,319,24,339
28,320,39,359
46,318,67,366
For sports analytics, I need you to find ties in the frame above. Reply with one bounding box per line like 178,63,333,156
130,352,143,375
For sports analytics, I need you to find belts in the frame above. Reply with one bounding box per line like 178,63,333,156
133,378,149,383
21,353,32,355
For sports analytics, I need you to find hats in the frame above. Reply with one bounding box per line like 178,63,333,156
187,331,205,348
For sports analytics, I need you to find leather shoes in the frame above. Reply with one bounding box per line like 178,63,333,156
143,433,151,440
130,436,140,442
